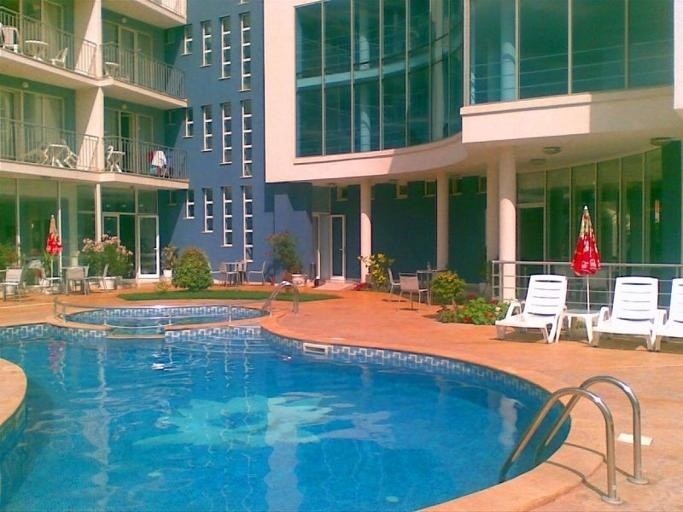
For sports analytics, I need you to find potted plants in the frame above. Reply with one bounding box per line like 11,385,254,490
266,232,305,286
78,236,133,290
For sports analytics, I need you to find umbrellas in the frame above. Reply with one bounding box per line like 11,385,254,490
45,212,63,288
567,204,601,314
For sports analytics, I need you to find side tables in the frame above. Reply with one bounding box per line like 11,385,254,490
555,309,600,343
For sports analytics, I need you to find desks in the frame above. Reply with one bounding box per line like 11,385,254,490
417,269,435,289
25,40,49,62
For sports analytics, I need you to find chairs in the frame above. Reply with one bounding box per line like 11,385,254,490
105,146,114,170
0,27,21,54
655,278,683,352
593,277,667,351
49,47,68,68
219,261,266,287
387,268,429,312
40,264,109,295
1,267,24,301
495,275,567,343
